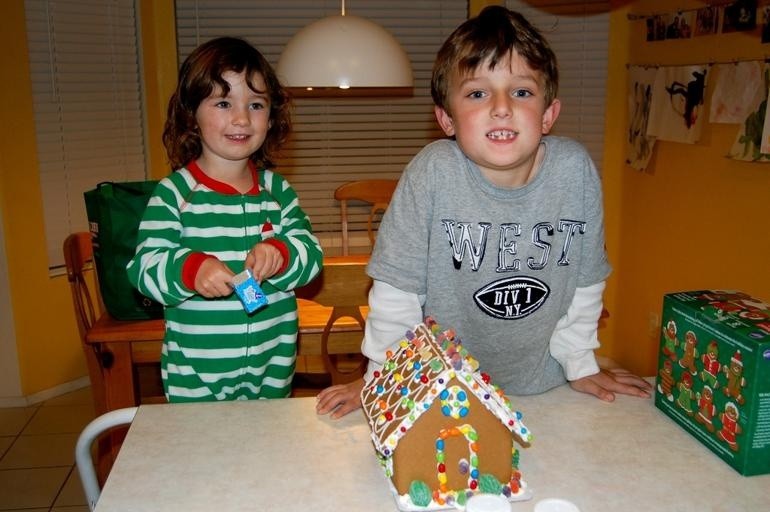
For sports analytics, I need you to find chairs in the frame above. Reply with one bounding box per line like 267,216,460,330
279,264,377,396
335,178,398,258
62,231,168,492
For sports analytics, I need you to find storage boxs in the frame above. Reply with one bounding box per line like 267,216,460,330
653,289,770,476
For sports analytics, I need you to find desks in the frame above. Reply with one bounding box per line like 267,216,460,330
89,375,770,512
84,234,611,465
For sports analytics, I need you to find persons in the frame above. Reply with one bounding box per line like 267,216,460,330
124,34,324,403
312,3,655,417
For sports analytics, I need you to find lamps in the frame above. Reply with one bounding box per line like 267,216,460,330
273,0,414,99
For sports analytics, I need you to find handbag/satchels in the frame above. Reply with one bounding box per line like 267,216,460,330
83,180,163,320
